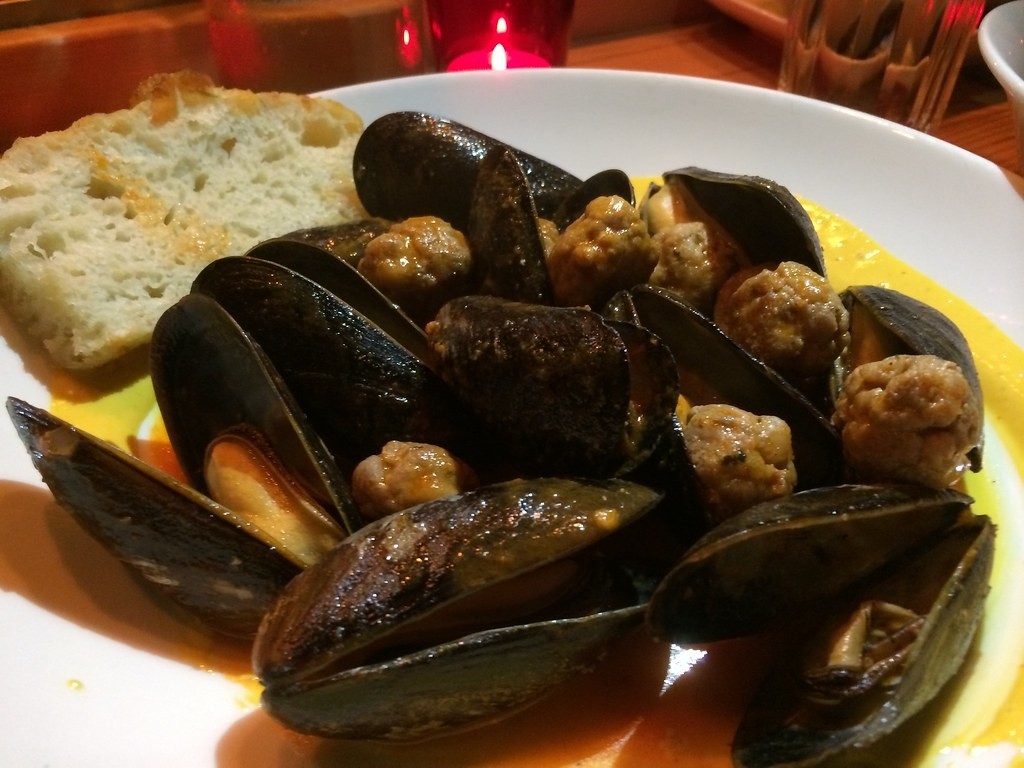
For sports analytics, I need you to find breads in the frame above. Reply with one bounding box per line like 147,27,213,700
0,70,366,371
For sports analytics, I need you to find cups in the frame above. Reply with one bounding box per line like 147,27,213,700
420,0,578,74
776,0,985,138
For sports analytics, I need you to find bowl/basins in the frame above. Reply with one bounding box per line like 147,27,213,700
976,0,1024,161
0,64,1024,768
